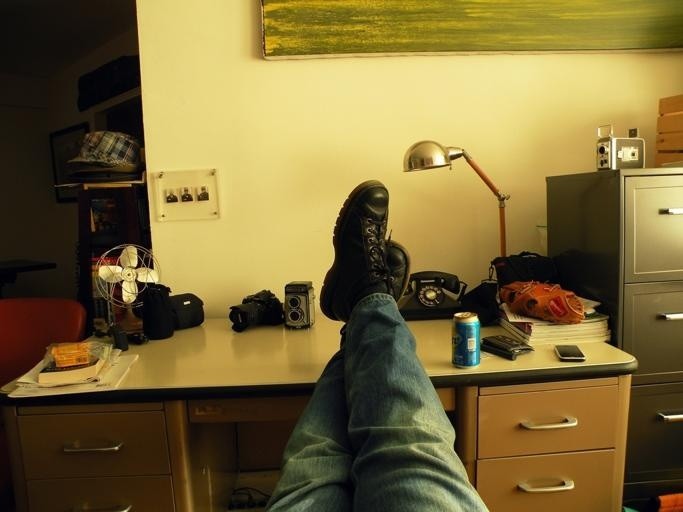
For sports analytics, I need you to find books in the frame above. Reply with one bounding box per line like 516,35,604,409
498,295,610,347
38,345,105,384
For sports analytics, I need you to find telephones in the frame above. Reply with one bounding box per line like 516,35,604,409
398,270,467,320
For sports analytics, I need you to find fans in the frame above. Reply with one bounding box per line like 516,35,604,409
95,244,162,336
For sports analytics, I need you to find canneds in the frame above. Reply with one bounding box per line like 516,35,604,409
451,312,481,368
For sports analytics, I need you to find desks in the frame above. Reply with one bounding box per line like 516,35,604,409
0,259,57,297
1,316,639,512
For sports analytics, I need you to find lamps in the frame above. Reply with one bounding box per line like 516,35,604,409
404,141,510,258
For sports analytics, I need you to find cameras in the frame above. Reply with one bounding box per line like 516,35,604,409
227,288,284,331
284,281,316,330
142,281,206,339
595,123,646,170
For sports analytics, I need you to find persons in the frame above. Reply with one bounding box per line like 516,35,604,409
166,189,177,201
263,180,490,511
197,186,208,200
180,187,193,201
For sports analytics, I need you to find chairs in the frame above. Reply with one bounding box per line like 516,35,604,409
0,297,87,388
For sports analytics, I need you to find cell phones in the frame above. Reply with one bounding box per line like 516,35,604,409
555,342,587,360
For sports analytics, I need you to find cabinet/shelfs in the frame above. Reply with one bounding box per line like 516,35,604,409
545,167,683,512
82,86,146,191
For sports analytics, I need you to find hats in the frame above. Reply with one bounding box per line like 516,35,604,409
66,130,141,169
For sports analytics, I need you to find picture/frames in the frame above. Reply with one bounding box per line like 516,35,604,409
259,0,683,61
49,121,89,203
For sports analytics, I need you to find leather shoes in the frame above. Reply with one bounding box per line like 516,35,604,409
320,179,411,349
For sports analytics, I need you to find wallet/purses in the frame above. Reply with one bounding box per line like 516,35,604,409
481,336,533,360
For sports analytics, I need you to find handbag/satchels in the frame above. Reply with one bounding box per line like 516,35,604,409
493,251,557,302
